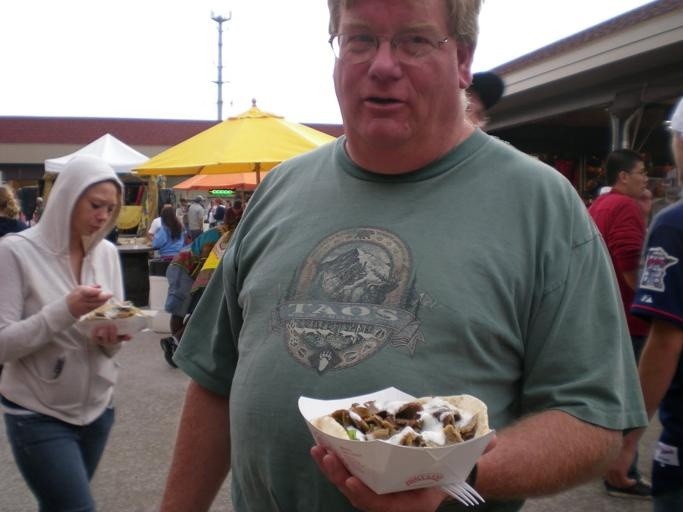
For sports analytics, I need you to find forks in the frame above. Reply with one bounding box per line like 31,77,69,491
441,481,485,507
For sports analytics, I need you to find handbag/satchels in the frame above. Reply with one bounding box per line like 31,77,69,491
214,206,225,220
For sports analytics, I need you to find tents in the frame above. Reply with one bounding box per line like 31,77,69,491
44,132,150,173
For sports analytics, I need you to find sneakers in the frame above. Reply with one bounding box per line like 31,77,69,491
161,337,178,368
604,476,653,500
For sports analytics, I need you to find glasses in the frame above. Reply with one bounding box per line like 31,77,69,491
329,30,457,65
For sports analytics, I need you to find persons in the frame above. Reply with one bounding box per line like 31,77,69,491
589,96,683,512
1,181,247,367
466,73,505,130
0,155,132,512
162,1,650,511
